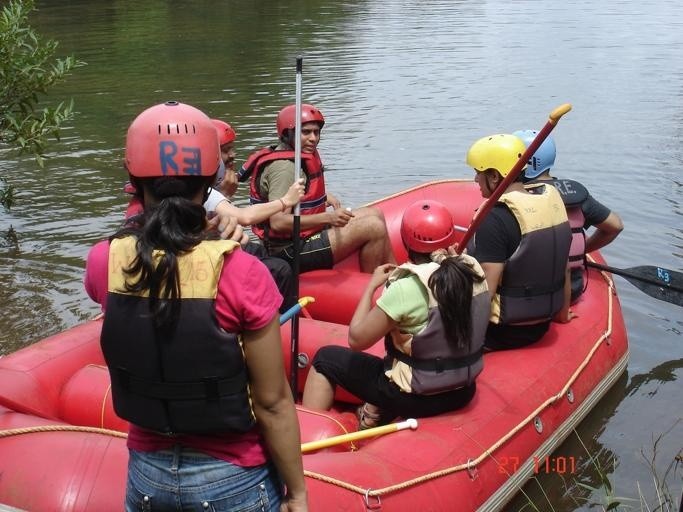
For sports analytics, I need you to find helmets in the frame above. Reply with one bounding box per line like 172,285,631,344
277,104,325,140
400,199,455,254
125,101,236,177
465,129,556,179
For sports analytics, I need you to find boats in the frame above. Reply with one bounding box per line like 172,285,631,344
1,172,631,511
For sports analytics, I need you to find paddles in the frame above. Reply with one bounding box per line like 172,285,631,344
454,225,682,307
289,55,302,405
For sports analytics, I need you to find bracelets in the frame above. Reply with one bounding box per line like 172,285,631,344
279,198,286,213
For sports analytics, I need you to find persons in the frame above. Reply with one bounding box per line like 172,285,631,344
247,104,401,277
80,98,310,511
446,134,582,353
301,196,492,435
512,130,625,309
200,115,306,313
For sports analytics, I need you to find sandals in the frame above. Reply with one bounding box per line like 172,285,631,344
355,402,380,431
